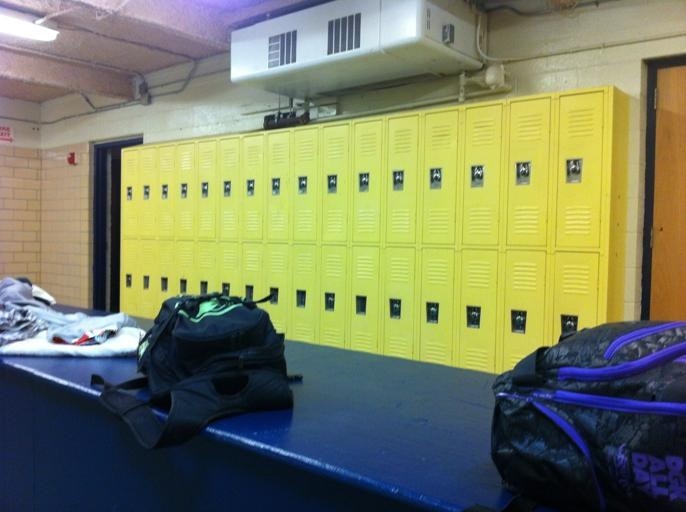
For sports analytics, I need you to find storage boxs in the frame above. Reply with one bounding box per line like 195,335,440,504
0,302,510,511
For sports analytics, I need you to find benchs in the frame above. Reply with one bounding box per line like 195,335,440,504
0,302,510,511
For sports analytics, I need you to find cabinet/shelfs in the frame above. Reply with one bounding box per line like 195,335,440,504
173,135,242,299
121,141,174,318
501,85,625,370
320,98,504,373
242,125,320,344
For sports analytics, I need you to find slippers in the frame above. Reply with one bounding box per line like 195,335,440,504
90,294,294,449
491,320,686,512
